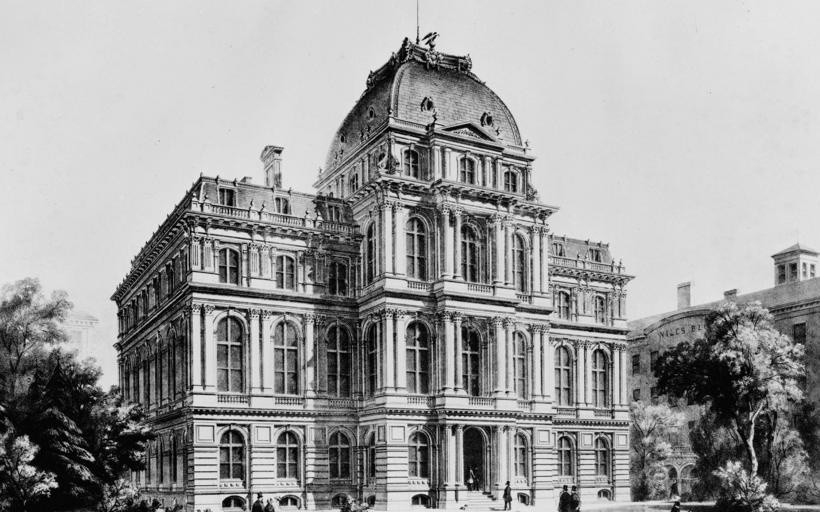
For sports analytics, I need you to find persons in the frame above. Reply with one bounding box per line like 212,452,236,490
253,465,582,511
670,501,680,512
